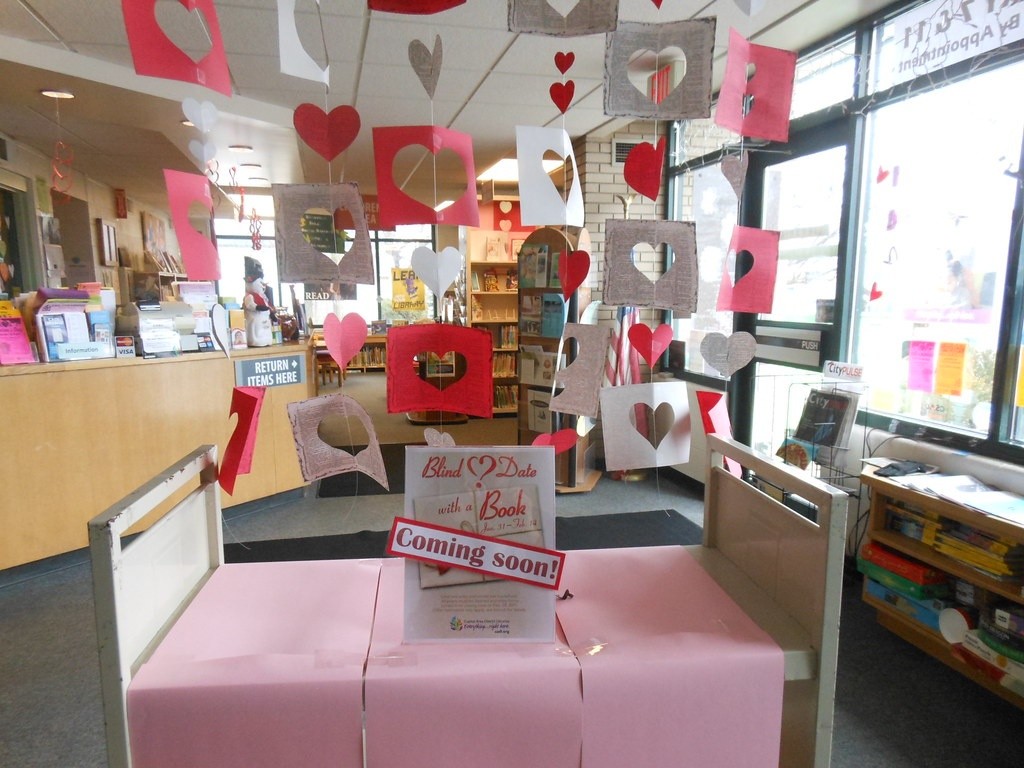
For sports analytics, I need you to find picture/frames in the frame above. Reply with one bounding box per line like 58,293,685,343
100,218,120,267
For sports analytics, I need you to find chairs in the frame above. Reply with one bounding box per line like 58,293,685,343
315,336,347,388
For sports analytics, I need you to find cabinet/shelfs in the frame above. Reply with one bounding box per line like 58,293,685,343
856,463,1024,712
313,333,388,373
133,270,188,304
463,231,536,419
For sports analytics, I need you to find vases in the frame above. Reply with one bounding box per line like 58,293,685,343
276,307,297,342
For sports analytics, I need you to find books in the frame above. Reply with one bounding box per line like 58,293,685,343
471,267,518,292
951,605,1024,698
517,244,570,434
372,320,386,335
885,498,1024,584
392,320,409,326
858,543,955,629
494,385,519,408
493,352,515,375
497,324,518,349
487,236,508,262
348,345,386,367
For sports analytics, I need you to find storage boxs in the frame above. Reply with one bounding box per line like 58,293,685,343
856,543,1024,681
